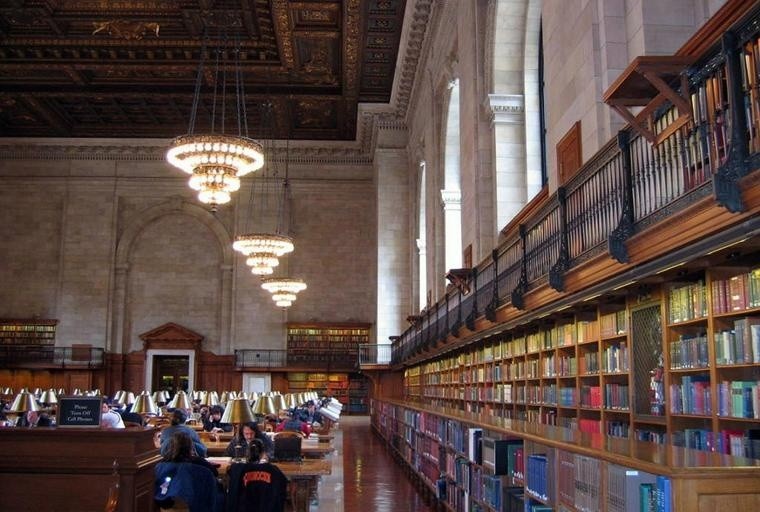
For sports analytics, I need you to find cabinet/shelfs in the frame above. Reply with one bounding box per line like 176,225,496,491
0,316,57,359
370,395,760,512
658,265,716,455
707,251,760,451
284,320,371,417
402,302,632,436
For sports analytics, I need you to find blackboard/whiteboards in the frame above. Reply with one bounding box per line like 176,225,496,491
56,394,104,429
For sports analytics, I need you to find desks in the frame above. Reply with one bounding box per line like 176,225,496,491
154,430,335,512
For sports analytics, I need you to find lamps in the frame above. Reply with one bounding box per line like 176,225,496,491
1,388,339,429
229,75,296,283
163,1,265,211
258,114,309,313
219,397,258,465
